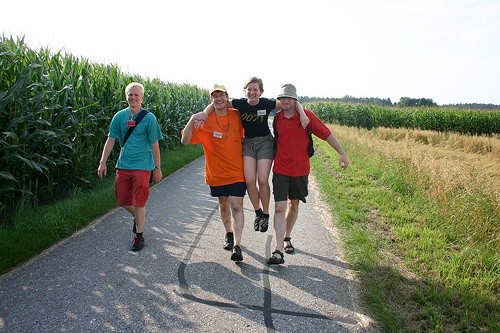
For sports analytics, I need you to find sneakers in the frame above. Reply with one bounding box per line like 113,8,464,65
131,236,144,251
224,231,234,251
133,213,146,234
231,245,243,261
254,208,269,232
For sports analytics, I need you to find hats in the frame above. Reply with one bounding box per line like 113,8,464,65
209,83,227,94
277,84,299,101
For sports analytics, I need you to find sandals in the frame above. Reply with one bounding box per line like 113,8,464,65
267,250,285,264
282,237,294,253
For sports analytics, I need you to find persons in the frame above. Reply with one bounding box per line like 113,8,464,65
97,82,163,251
182,77,245,264
194,77,310,233
267,84,349,265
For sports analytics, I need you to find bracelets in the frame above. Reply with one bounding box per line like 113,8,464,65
154,166,162,169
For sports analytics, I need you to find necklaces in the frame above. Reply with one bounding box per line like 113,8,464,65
214,108,230,138
